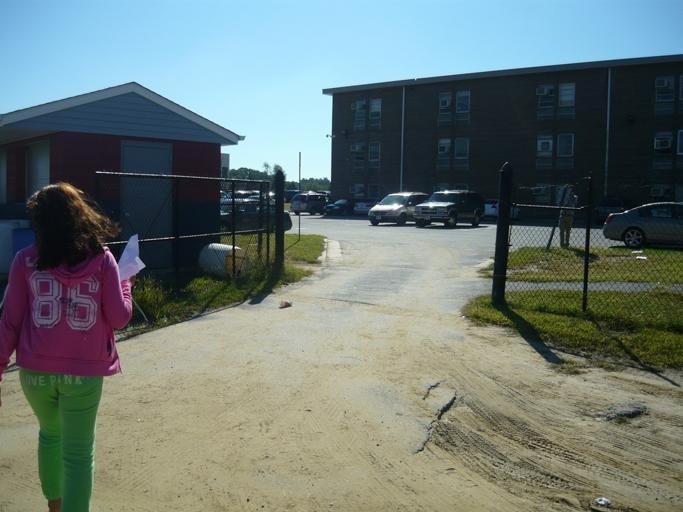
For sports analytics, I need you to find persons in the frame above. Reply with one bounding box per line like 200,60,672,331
0,181,136,512
559,185,578,248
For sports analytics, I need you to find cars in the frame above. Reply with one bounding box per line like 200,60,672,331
599,201,682,249
323,191,517,229
220,188,329,233
